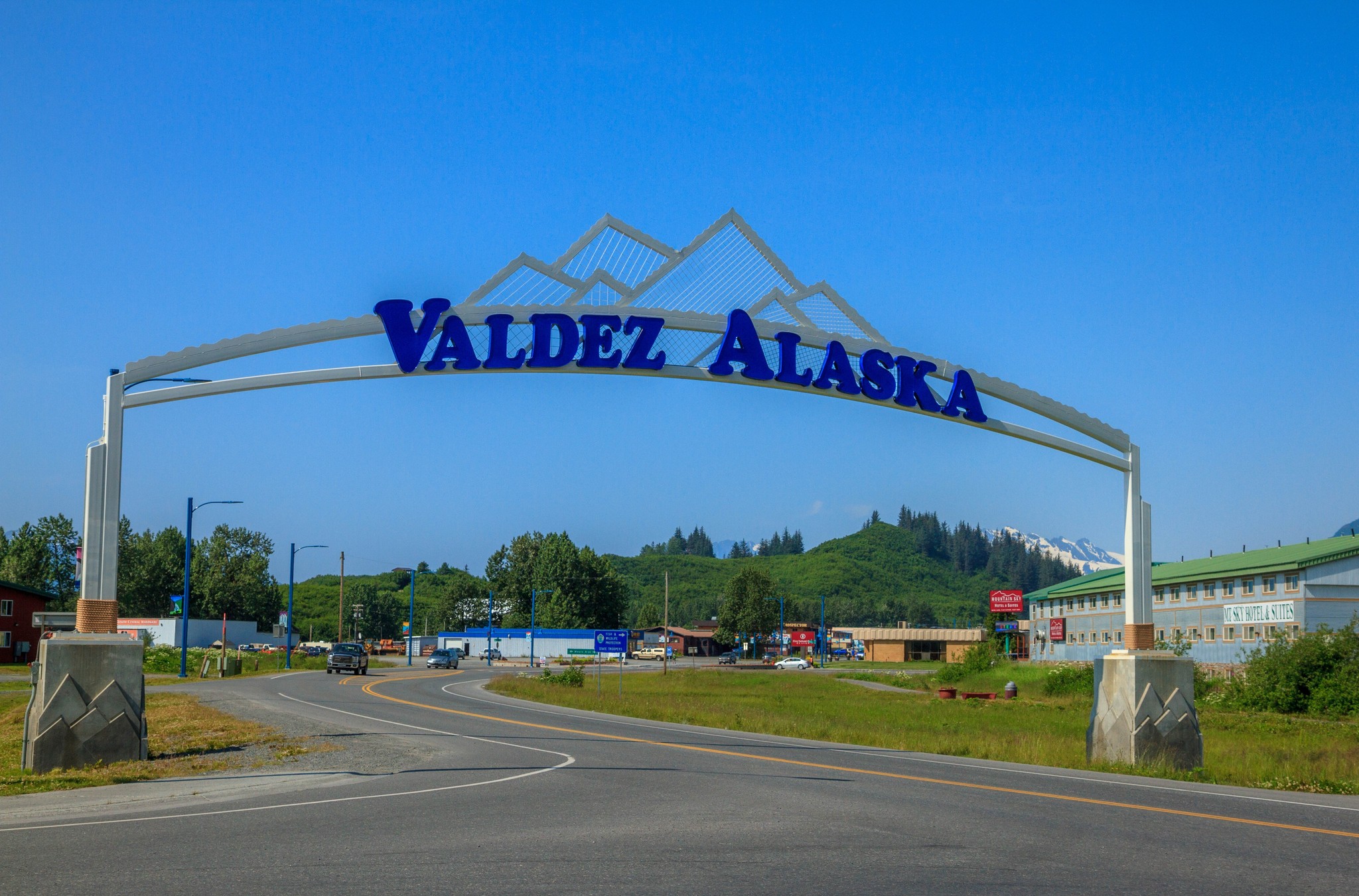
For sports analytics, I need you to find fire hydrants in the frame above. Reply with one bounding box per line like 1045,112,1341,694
772,661,774,665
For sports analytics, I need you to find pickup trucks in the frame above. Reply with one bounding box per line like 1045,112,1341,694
479,648,502,660
631,648,664,661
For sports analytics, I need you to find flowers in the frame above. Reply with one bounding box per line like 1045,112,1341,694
940,686,957,690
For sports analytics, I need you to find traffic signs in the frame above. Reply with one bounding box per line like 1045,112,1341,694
567,630,628,655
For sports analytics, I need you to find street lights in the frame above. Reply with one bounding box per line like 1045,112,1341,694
531,589,554,667
407,569,439,667
739,630,777,660
178,498,243,677
846,633,854,648
839,636,845,656
284,543,329,669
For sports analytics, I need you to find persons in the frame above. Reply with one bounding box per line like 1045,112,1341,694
672,652,676,664
810,656,814,668
851,649,855,660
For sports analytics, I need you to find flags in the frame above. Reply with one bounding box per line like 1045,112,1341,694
279,612,287,626
169,596,182,616
403,622,409,634
526,632,531,642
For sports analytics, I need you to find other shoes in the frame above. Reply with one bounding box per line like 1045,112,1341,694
675,662,676,664
673,662,674,664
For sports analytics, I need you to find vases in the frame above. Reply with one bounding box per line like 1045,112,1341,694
961,692,997,700
938,689,957,699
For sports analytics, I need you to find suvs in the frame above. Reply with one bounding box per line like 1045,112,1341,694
426,648,465,669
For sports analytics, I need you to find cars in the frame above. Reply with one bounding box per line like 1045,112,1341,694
832,648,853,655
762,652,777,661
643,647,660,652
718,652,736,664
774,658,811,670
732,647,744,656
240,642,369,675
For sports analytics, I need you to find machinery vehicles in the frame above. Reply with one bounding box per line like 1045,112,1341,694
363,638,378,655
